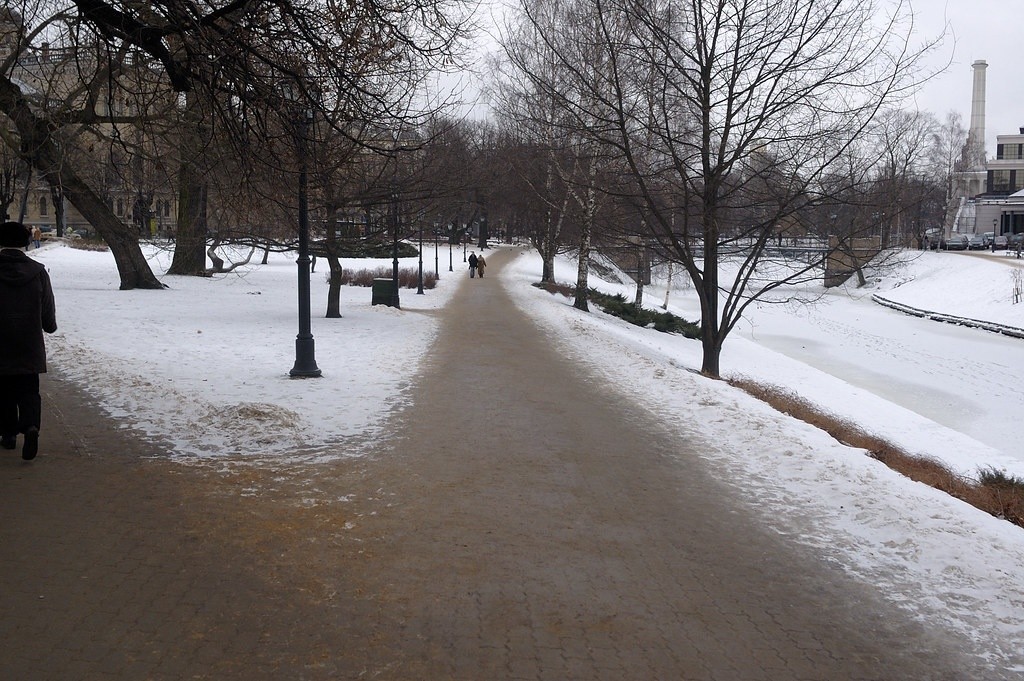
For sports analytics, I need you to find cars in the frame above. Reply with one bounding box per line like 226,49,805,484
946,234,969,251
984,232,1024,251
969,238,984,250
974,234,990,249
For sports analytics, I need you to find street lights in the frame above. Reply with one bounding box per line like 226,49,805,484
462,222,467,263
388,175,401,310
480,216,485,251
416,209,424,294
280,61,322,379
992,218,998,252
447,222,454,271
433,220,439,280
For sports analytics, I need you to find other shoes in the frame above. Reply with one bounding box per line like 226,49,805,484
0,436,16,450
36,246,39,248
471,276,474,278
479,275,483,278
22,428,38,461
26,247,29,251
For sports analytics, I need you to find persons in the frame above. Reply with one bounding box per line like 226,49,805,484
25,224,41,251
778,230,782,247
1,221,57,460
167,227,175,243
1016,242,1021,259
468,251,486,278
917,236,929,250
67,226,72,234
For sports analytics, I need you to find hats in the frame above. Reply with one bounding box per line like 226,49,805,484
0,222,30,248
